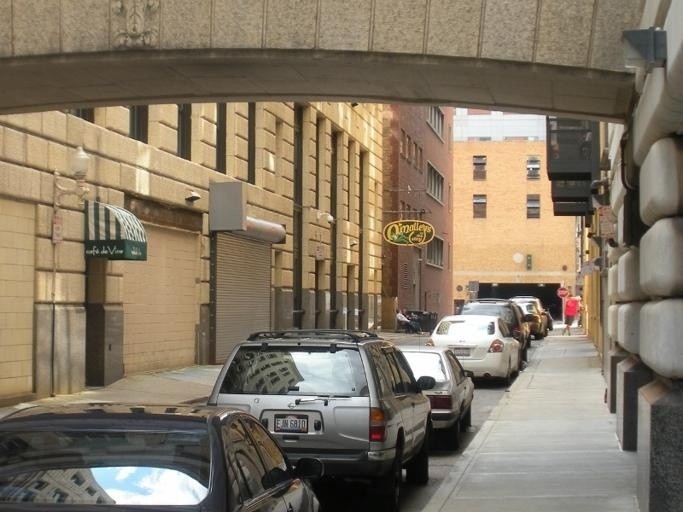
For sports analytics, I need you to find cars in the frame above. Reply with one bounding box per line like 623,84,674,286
508,296,553,340
396,345,476,452
1,398,326,511
424,314,522,388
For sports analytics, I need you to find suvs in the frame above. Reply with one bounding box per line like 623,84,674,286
206,327,433,512
457,298,536,372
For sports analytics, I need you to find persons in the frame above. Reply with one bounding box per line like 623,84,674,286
395,308,423,336
402,308,422,332
561,285,582,336
